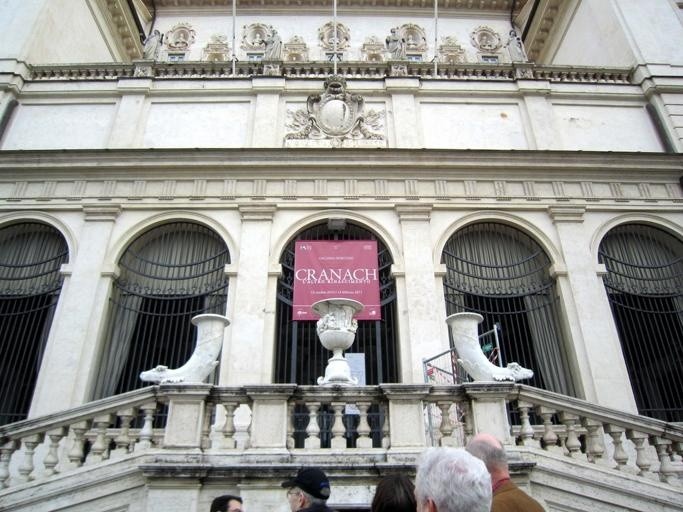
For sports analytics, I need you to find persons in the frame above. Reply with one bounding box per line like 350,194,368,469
281,468,338,511
413,444,494,512
464,434,547,512
209,495,244,511
370,475,416,512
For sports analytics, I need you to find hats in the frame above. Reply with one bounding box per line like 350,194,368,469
280,466,331,500
